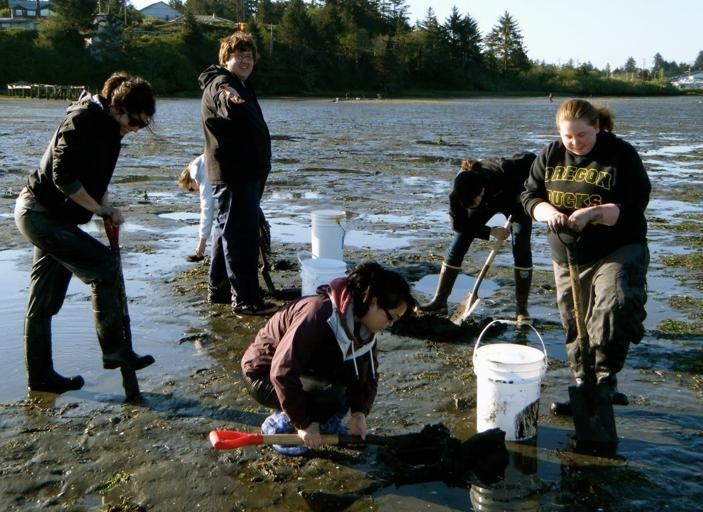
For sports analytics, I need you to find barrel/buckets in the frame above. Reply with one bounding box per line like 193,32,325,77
296,250,347,296
473,320,547,443
310,210,345,259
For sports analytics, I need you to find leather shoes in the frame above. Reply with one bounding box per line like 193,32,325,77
551,380,629,414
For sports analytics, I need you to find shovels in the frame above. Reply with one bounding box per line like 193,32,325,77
555,224,618,442
258,232,275,295
208,428,435,454
103,214,140,398
449,214,515,326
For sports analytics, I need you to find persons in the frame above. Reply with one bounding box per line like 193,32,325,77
194,30,282,317
176,151,277,265
415,147,537,330
12,68,162,395
232,257,416,455
516,97,654,420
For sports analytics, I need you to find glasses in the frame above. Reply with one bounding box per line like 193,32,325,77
124,108,150,128
189,182,194,191
384,309,400,322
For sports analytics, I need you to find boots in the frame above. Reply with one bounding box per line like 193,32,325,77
419,262,462,312
24,315,84,394
514,267,534,328
94,308,155,370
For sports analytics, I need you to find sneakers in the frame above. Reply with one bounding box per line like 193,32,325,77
261,411,308,455
208,295,278,314
319,416,350,434
